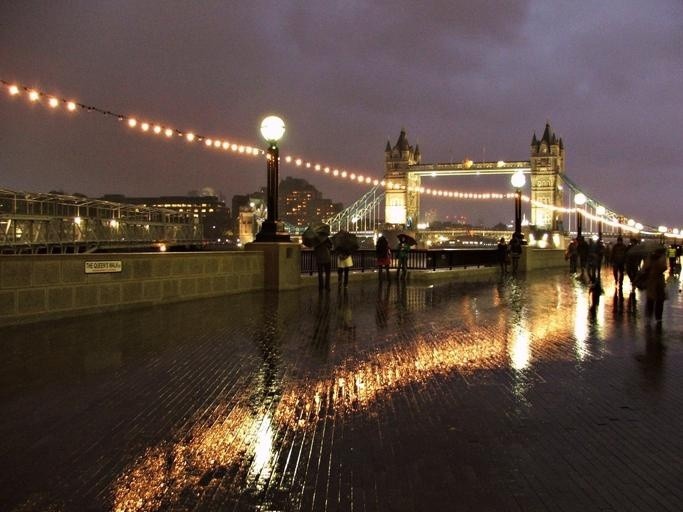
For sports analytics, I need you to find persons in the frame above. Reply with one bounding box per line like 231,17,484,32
315,237,331,293
509,232,522,274
337,253,354,289
564,235,683,325
395,237,410,281
497,237,510,274
377,248,391,280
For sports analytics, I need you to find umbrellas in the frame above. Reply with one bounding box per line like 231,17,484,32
375,236,388,258
332,229,360,256
302,222,331,248
397,233,416,243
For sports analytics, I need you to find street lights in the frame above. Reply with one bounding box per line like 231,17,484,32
595,205,605,239
256,112,291,243
511,171,529,245
574,192,586,238
